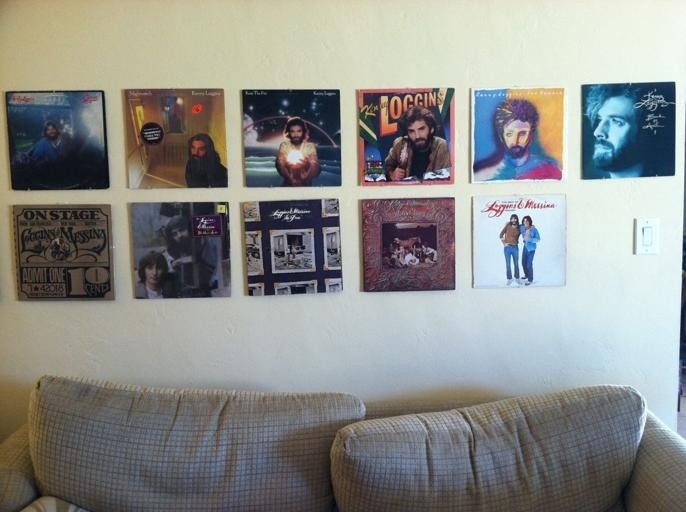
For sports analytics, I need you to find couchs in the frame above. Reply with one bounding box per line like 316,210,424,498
0,383,685,512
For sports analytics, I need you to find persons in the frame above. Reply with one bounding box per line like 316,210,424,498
519,216,541,285
384,106,452,181
499,214,521,286
275,118,321,186
185,133,228,188
135,216,216,298
474,99,562,181
586,84,651,177
21,119,74,162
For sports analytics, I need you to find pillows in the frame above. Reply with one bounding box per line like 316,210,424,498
28,372,365,511
330,384,646,511
